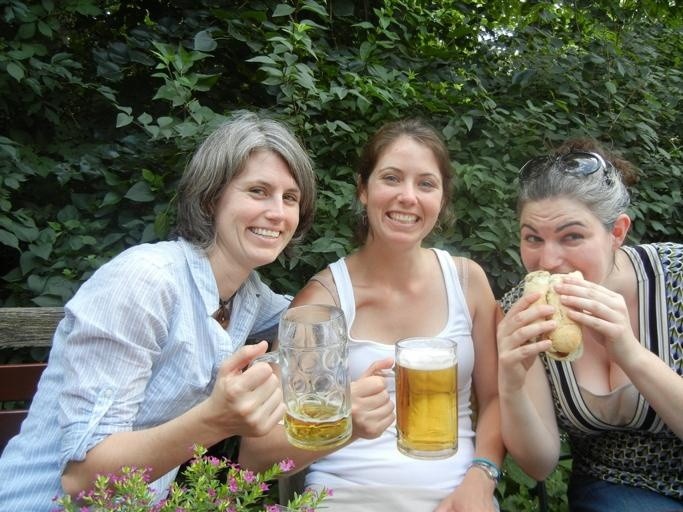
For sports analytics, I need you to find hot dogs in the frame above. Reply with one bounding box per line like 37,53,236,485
524,270,583,361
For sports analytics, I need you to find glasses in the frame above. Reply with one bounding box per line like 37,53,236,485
516,151,613,188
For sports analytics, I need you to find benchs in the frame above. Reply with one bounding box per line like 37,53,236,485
0,364,65,456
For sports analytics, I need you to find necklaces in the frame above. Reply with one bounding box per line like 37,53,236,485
215,292,236,329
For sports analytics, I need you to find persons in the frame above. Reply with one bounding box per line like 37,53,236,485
232,116,510,511
0,108,318,512
490,136,683,511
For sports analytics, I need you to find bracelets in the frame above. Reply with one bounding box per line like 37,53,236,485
468,458,501,486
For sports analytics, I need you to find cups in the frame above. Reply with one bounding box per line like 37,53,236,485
371,338,458,461
248,305,353,452
